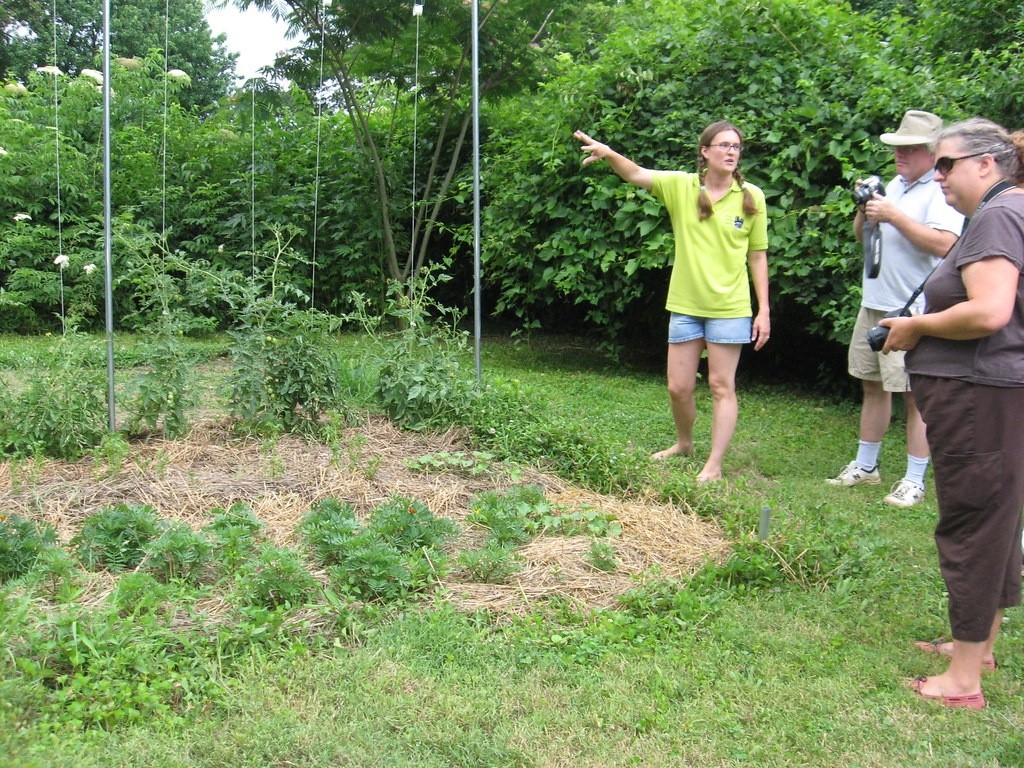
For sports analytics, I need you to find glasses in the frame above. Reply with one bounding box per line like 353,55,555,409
891,145,927,153
708,142,744,152
935,151,998,175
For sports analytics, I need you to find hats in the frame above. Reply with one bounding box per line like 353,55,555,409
879,110,943,146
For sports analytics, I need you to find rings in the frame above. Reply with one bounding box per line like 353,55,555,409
767,337,770,339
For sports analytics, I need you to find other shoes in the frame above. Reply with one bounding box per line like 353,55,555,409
913,640,994,674
902,677,984,710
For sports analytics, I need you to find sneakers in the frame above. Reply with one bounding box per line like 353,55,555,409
825,461,881,489
884,479,925,509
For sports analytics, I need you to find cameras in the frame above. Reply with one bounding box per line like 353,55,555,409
867,308,911,352
850,178,886,206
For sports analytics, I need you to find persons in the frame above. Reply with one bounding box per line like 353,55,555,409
573,122,771,487
877,119,1024,708
827,110,968,508
